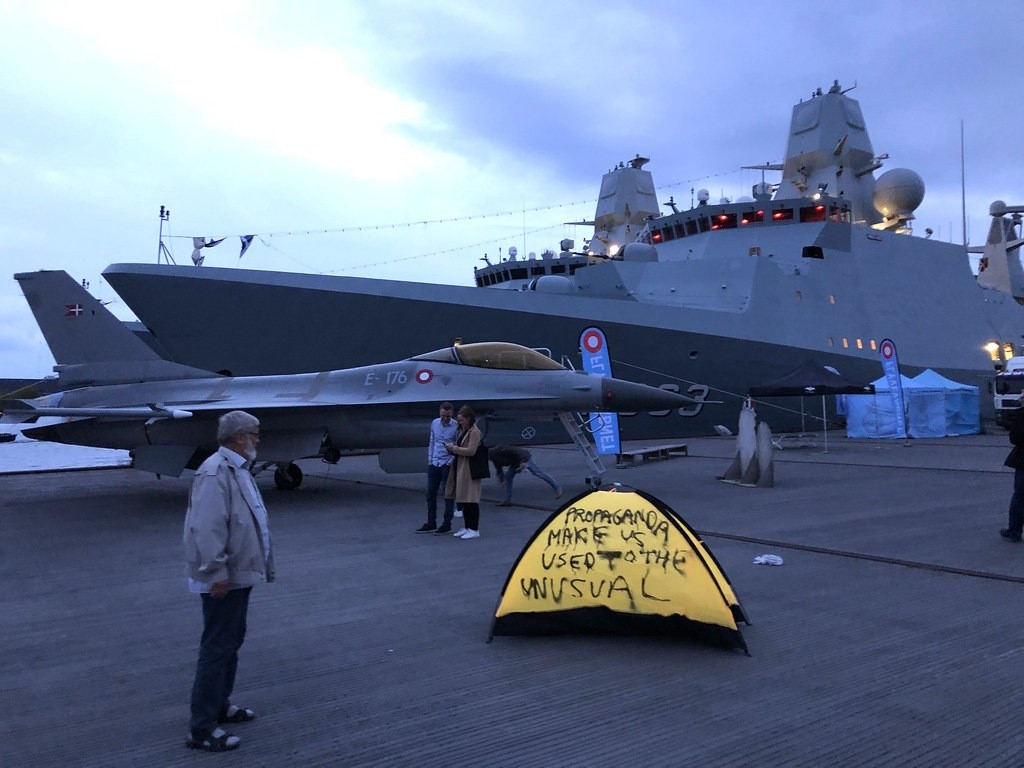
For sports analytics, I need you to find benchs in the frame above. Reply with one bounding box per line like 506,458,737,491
614,444,687,465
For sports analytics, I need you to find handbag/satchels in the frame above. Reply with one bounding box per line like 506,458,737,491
468,428,491,481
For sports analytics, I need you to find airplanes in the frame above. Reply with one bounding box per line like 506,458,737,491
15,268,725,491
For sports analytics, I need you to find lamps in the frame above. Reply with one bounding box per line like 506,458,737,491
819,182,830,196
925,228,934,238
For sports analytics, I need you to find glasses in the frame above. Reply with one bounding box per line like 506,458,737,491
242,430,259,438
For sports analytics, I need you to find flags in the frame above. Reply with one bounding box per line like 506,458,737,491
193,236,206,249
191,248,200,265
195,256,205,266
205,239,224,247
240,235,254,258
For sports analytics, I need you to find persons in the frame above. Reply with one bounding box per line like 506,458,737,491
488,444,563,506
444,405,484,539
415,402,459,535
184,410,275,751
1000,396,1024,543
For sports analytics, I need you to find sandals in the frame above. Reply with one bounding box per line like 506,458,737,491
218,705,256,721
185,727,240,752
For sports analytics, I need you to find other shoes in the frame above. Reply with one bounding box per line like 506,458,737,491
1000,528,1022,543
555,486,563,499
496,500,512,507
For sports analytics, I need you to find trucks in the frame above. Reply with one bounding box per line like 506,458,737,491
992,355,1024,428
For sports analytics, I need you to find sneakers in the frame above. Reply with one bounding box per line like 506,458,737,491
416,523,438,533
462,528,480,539
453,528,467,536
434,524,452,535
453,510,463,517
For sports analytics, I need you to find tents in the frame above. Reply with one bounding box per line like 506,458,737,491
487,482,751,655
845,369,980,439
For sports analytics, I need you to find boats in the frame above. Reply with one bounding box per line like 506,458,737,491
123,81,1024,437
0,432,17,443
